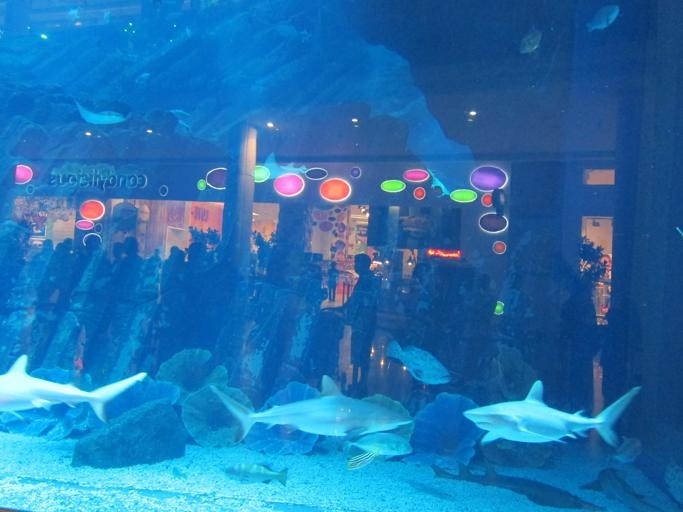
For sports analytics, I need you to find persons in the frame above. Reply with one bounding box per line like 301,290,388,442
0,218,624,440
